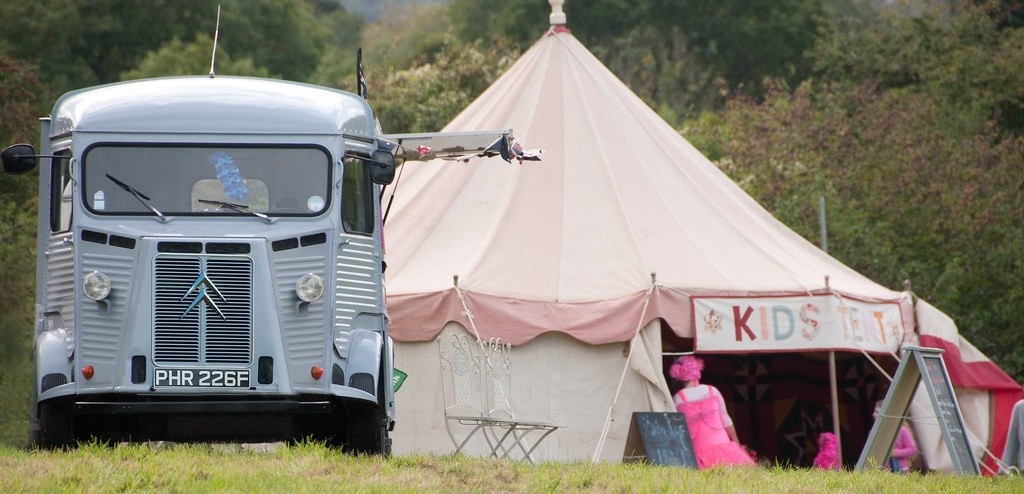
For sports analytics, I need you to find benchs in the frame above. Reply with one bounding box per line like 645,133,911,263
436,333,569,466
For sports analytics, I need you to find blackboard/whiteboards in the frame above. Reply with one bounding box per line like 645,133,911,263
851,347,982,480
622,411,698,471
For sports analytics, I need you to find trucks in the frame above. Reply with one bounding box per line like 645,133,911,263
2,70,542,462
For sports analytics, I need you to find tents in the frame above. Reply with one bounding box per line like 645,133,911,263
383,0,1023,474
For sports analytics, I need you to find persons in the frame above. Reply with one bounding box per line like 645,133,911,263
670,357,754,469
998,400,1023,471
868,397,915,473
